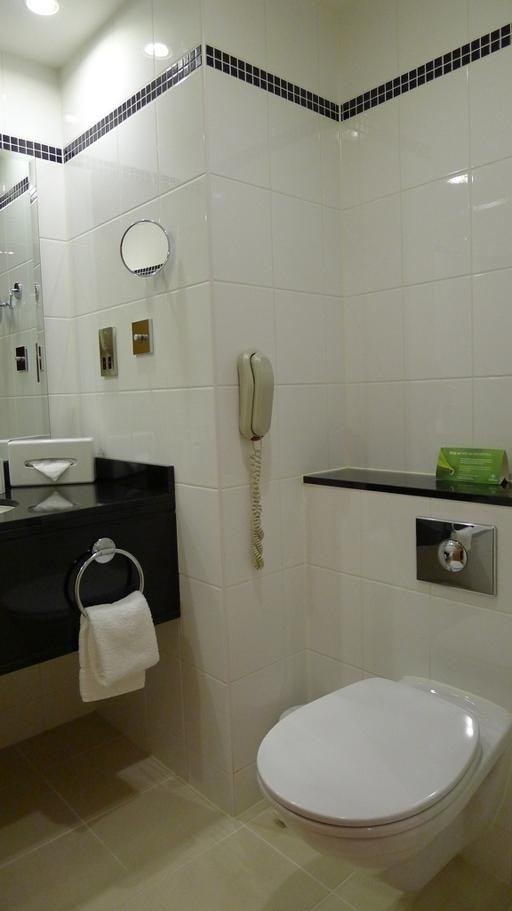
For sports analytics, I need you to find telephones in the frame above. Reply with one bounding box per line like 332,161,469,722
237,350,276,451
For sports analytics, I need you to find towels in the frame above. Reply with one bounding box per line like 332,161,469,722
76,589,161,705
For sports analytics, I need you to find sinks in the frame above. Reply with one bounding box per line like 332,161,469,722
0,502,16,513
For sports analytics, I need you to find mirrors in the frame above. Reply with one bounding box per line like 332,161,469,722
0,147,51,474
117,218,169,278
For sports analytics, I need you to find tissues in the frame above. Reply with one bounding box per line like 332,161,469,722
11,484,100,515
7,436,96,487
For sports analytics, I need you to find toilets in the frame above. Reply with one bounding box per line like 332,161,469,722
252,672,512,901
